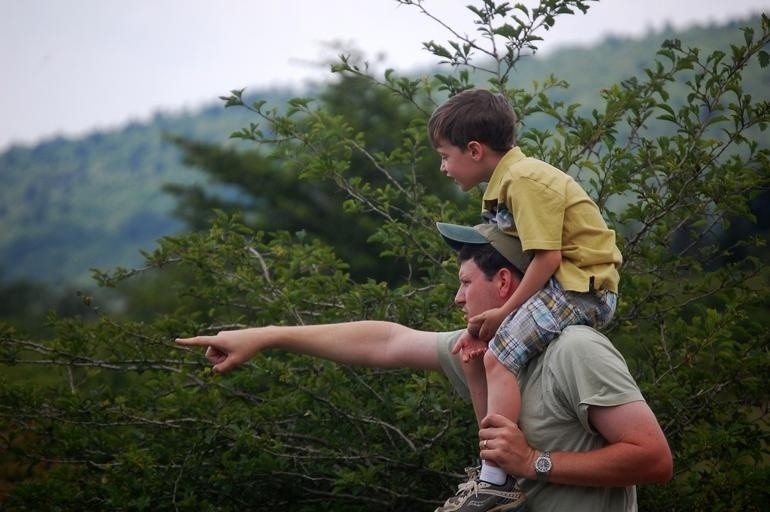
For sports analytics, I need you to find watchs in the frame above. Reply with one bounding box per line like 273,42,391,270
533,449,552,484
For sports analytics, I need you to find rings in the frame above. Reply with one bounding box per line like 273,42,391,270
484,440,488,448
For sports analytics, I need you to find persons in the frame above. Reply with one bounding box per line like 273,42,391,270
175,221,674,512
426,89,622,512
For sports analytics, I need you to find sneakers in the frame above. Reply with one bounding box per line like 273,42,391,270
433,463,528,512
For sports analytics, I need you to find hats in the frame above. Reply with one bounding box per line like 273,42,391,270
436,220,534,275
